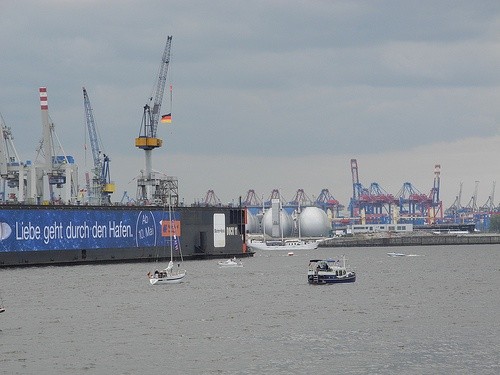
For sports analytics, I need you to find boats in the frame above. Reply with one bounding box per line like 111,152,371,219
387,252,406,256
217,256,246,269
248,195,320,250
307,254,356,285
0,35,257,268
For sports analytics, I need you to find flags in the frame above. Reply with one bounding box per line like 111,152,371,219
159,113,172,124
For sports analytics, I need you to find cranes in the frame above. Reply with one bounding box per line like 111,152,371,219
481,181,495,212
82,87,114,206
449,182,462,209
465,181,480,211
136,35,172,203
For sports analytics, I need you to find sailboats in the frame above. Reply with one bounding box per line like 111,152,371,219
148,182,186,285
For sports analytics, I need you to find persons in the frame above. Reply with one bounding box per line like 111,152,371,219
325,264,329,271
154,269,159,278
316,265,321,268
